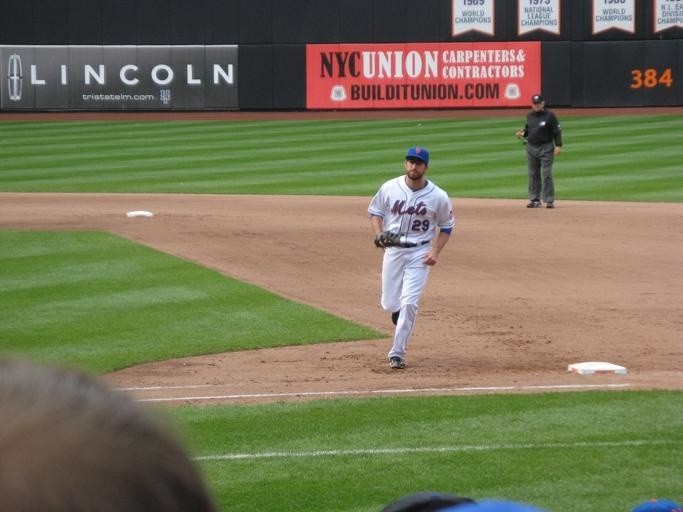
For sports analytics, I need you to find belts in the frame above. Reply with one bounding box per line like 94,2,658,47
399,240,429,248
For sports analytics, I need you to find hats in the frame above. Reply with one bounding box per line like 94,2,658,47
406,146,429,164
532,95,545,103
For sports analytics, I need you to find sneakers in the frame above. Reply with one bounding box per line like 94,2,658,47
392,312,399,324
390,356,405,368
546,202,553,208
527,201,541,208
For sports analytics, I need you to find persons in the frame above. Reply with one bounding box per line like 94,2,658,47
517,94,562,209
367,147,455,369
0,356,217,511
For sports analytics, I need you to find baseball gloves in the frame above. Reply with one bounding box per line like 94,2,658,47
375,232,403,248
